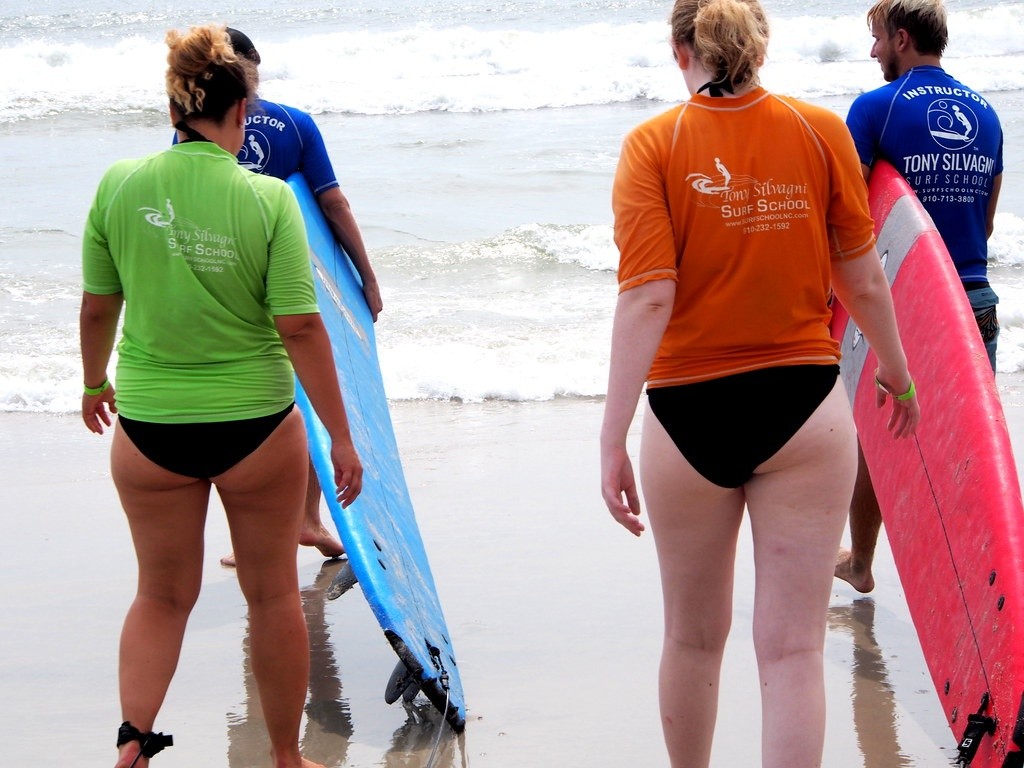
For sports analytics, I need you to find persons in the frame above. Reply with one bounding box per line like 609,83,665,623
600,0,921,768
833,0,1003,593
79,23,363,768
173,25,383,571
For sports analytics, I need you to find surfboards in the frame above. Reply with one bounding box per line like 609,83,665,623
259,169,467,734
827,157,1024,768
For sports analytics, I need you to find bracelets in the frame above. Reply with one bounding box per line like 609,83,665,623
874,368,915,400
82,376,109,396
